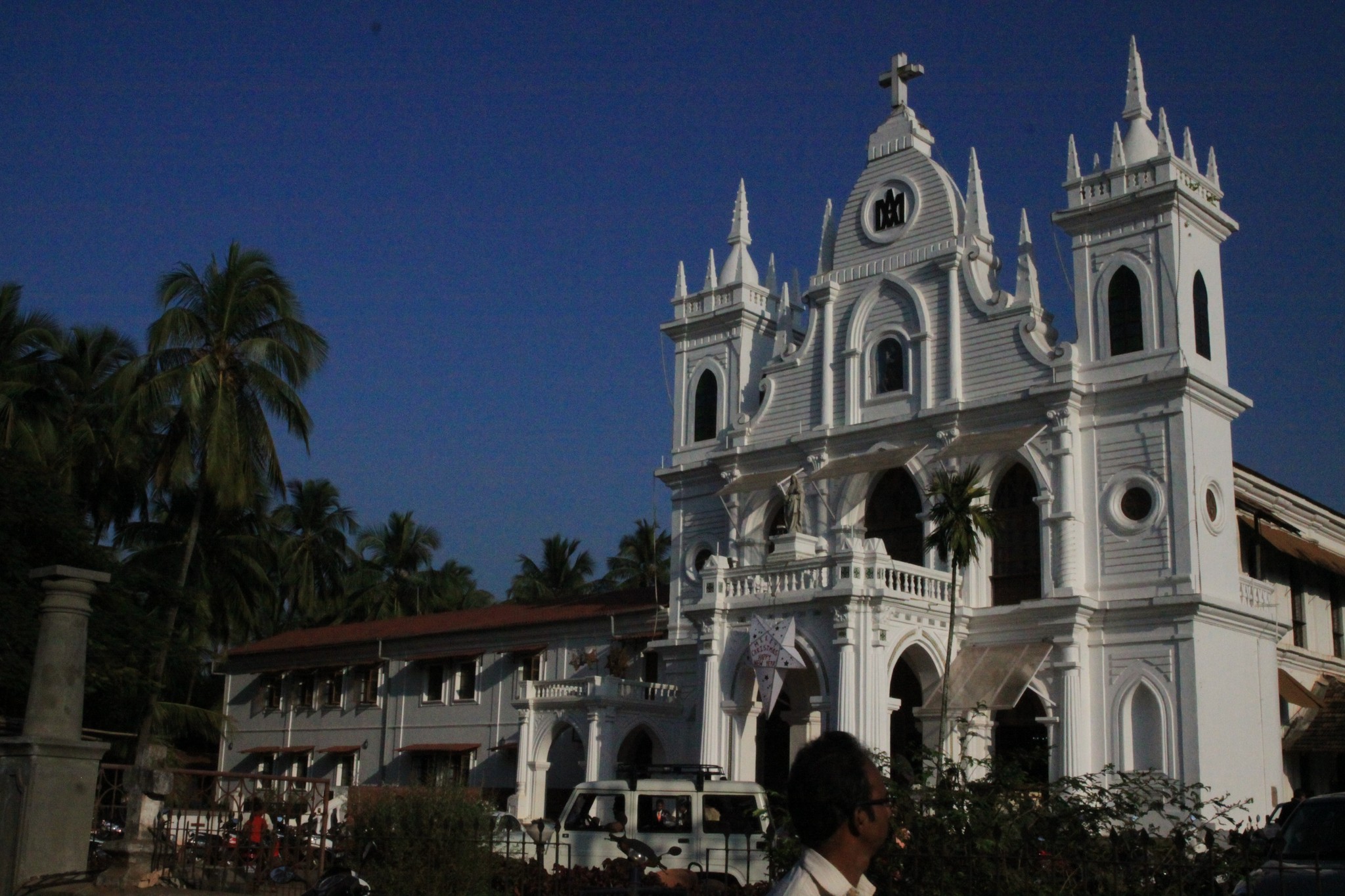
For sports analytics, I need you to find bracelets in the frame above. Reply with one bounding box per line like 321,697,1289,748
602,826,605,830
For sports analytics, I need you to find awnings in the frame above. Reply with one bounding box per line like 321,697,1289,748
1279,665,1345,707
1235,487,1345,573
911,640,1060,718
257,630,667,670
716,421,1052,538
242,740,519,753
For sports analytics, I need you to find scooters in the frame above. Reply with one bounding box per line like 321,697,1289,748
174,816,379,896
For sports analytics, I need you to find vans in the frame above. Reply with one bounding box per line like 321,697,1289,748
544,763,777,888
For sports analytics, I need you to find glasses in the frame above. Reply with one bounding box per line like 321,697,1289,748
859,791,895,808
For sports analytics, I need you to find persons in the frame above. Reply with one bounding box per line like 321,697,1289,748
243,803,275,845
765,730,894,896
648,798,690,826
782,473,805,532
599,796,626,833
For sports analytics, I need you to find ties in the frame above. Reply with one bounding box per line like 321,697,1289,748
657,812,661,821
